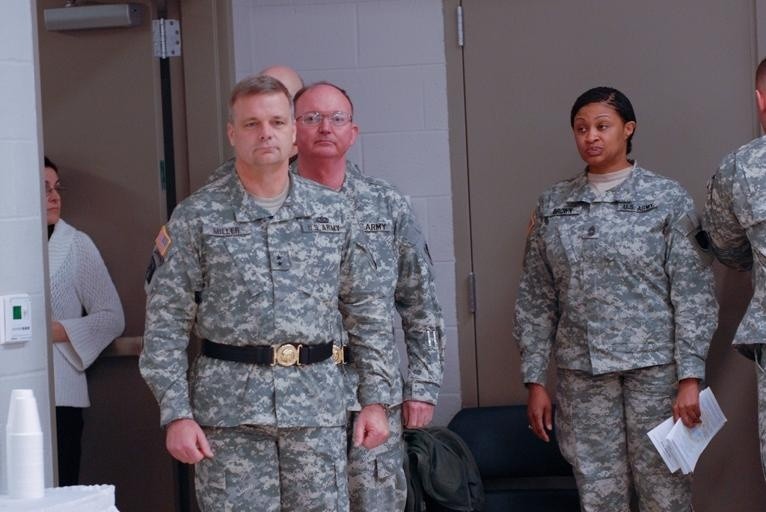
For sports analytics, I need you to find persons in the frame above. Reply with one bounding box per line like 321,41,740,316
700,58,766,481
204,63,306,179
511,84,721,511
284,80,445,512
42,154,128,487
138,76,404,512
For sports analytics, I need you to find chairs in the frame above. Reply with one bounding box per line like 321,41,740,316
447,405,581,512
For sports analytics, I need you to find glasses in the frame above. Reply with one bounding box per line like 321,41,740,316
296,111,352,127
46,183,67,195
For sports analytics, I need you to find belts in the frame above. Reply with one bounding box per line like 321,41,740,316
200,338,350,367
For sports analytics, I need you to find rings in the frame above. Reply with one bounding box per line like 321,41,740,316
527,421,533,432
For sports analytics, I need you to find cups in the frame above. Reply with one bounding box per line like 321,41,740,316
6,389,45,501
